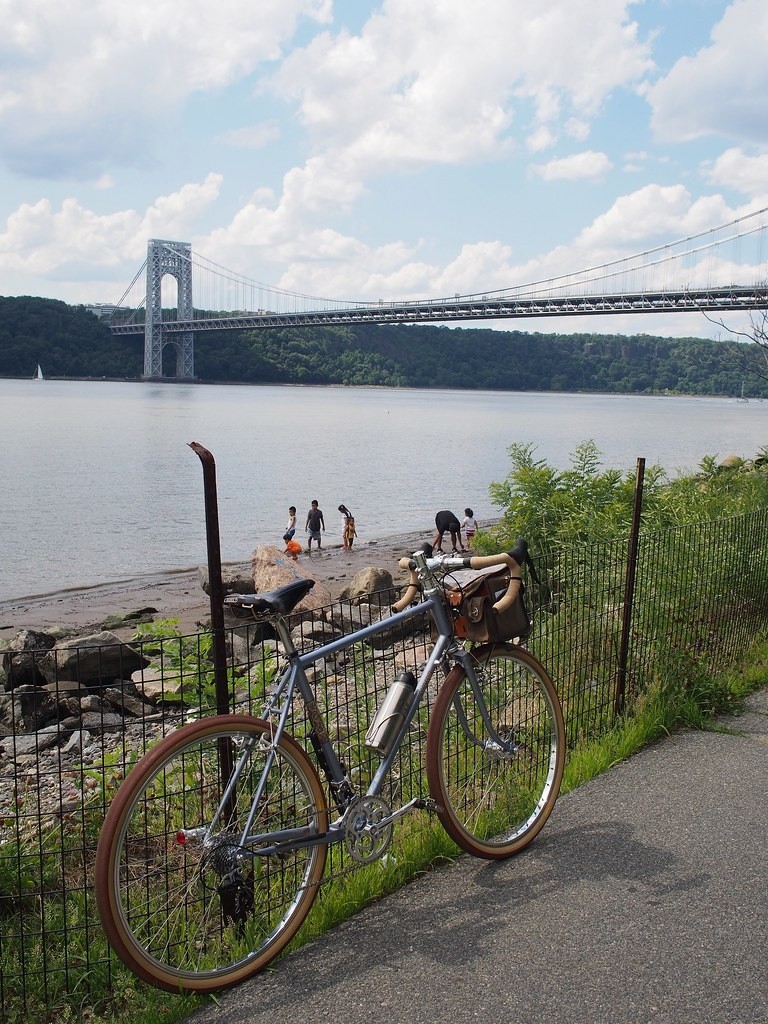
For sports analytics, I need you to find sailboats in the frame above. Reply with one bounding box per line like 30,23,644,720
31,363,45,381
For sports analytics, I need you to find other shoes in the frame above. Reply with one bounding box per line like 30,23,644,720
340,546,348,549
451,548,457,552
436,548,443,553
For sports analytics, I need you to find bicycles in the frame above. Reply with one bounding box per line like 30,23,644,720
95,542,565,996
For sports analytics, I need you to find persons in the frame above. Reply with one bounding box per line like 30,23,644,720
305,500,325,551
338,505,357,550
460,508,478,551
432,511,464,555
286,507,296,537
283,534,302,560
